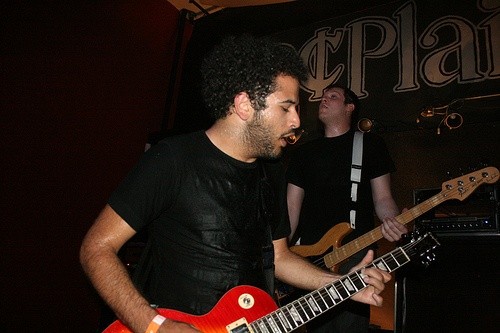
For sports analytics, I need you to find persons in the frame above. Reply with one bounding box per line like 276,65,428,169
79,26,391,333
282,82,406,333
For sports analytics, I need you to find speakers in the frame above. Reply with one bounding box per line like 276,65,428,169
394,232,500,333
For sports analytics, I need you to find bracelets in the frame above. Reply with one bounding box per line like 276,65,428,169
143,313,165,333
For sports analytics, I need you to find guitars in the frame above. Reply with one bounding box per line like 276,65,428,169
274,164,500,299
99,229,442,333
